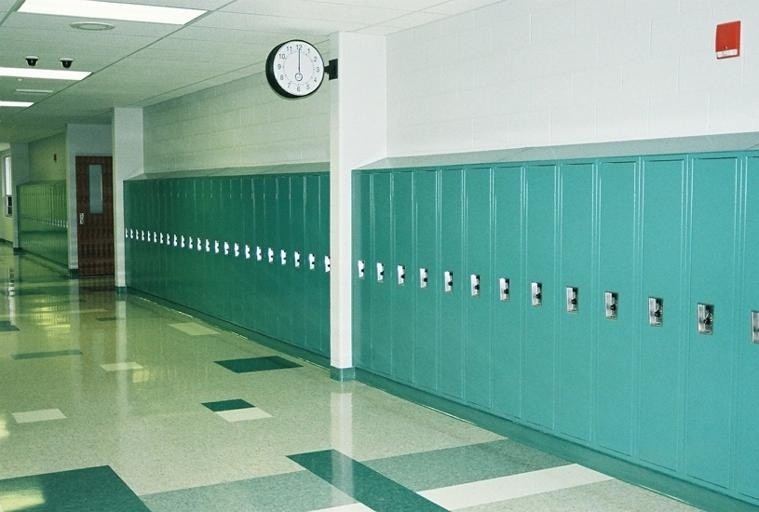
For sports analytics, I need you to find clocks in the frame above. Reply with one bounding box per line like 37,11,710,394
265,39,337,98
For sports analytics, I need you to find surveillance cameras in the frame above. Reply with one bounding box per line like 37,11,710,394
60,58,73,68
25,56,39,66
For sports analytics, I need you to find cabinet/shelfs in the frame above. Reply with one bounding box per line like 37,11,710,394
350,134,758,506
16,178,68,272
124,132,332,407
